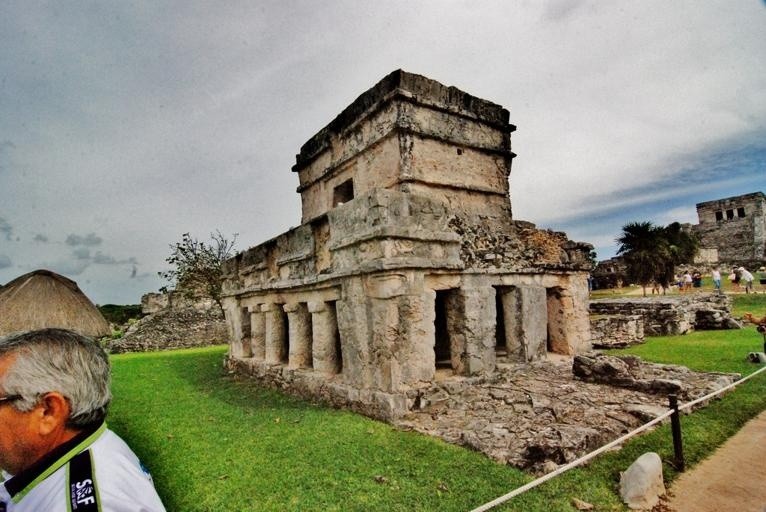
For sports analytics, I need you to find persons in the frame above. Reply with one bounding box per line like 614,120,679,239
588,276,594,297
0,326,165,512
650,264,766,295
743,310,766,355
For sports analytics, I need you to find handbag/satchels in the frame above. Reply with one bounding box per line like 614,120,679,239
727,270,736,281
